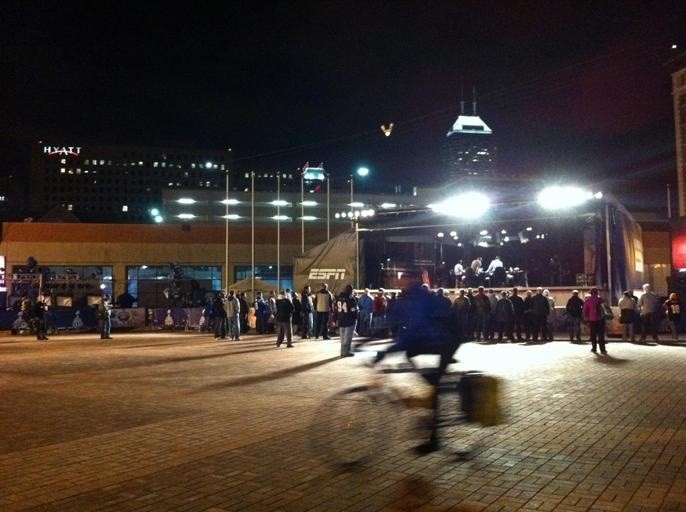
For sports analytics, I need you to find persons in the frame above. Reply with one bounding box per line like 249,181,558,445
471,256,483,275
275,291,295,347
254,283,397,340
16,293,31,322
189,290,250,341
484,255,504,276
335,285,359,357
453,258,467,282
368,268,463,455
34,295,49,340
566,284,686,353
98,293,113,339
422,284,556,342
434,259,451,286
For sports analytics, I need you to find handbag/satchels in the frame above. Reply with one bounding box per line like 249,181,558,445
599,303,614,320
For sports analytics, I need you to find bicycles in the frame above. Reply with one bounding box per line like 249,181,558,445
308,356,504,474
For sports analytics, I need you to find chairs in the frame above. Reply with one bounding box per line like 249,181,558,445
451,267,530,287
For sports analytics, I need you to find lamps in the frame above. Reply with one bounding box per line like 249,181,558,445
446,79,493,137
